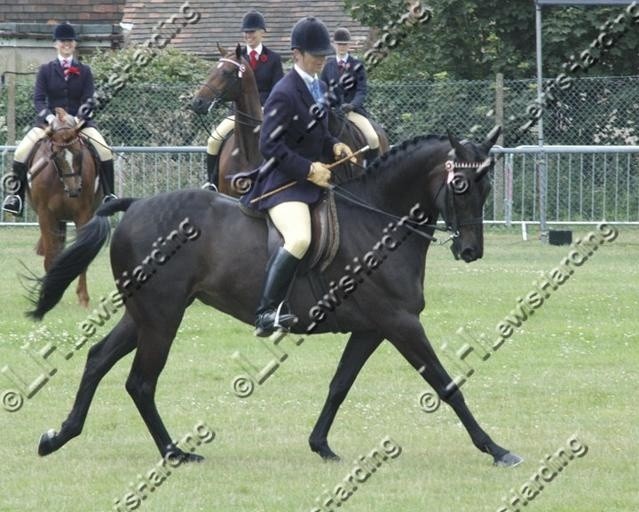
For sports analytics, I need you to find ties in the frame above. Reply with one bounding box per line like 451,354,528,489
249,50,257,68
63,60,70,81
311,79,322,103
338,61,345,74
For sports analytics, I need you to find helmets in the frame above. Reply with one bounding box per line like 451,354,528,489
240,11,266,31
53,23,78,41
290,17,336,57
334,27,352,44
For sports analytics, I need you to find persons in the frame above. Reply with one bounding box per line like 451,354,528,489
322,26,390,166
241,16,357,338
206,11,283,197
7,23,113,217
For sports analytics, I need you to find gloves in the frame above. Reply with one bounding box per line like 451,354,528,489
306,161,332,187
334,143,358,164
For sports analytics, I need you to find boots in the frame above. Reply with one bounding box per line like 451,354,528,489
3,161,27,217
254,246,300,338
98,159,115,205
206,152,216,184
366,146,379,167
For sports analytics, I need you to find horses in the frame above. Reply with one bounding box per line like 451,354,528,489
21,124,525,469
24,117,105,308
336,115,390,183
186,40,263,200
